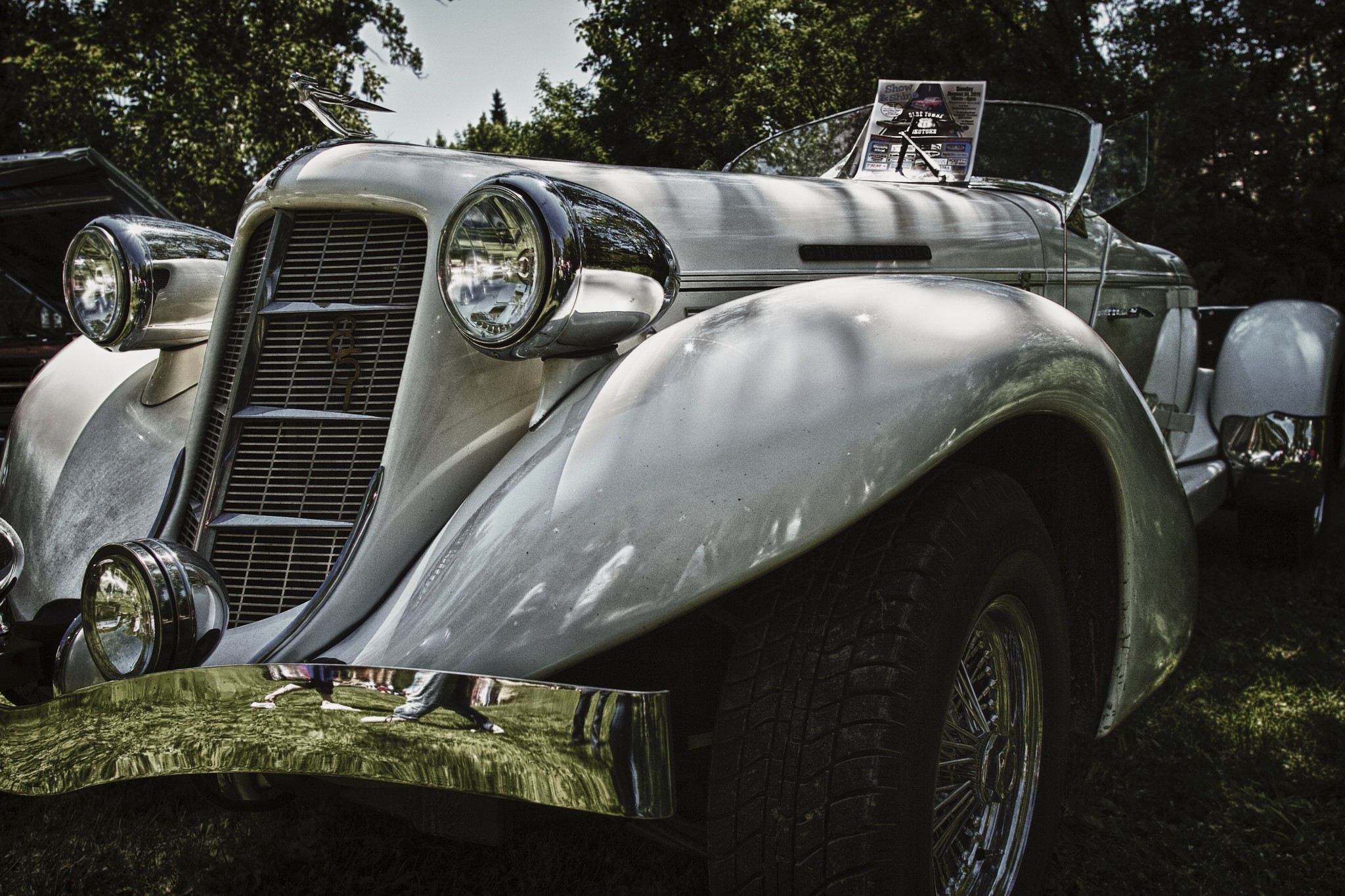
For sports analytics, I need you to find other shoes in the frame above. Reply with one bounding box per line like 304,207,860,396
321,703,362,712
360,714,409,723
334,678,402,697
250,702,276,708
491,724,505,734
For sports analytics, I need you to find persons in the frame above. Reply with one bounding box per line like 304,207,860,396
249,665,507,736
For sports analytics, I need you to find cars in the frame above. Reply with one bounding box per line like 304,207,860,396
1,78,1345,896
